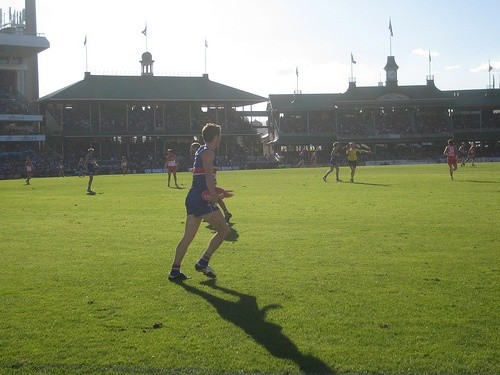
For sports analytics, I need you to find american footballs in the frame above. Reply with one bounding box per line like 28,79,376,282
201,187,225,203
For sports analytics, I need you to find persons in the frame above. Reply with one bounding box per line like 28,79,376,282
190,142,232,224
458,141,470,167
465,143,478,167
168,123,230,282
84,148,101,195
443,139,458,180
322,141,342,182
23,156,36,185
0,98,500,180
345,140,362,182
164,148,182,189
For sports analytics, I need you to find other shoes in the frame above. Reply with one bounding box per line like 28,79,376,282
87,189,96,194
323,176,328,182
350,177,354,182
336,178,342,182
225,213,232,223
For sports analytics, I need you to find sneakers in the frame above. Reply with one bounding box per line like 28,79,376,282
194,263,216,278
168,272,187,281
